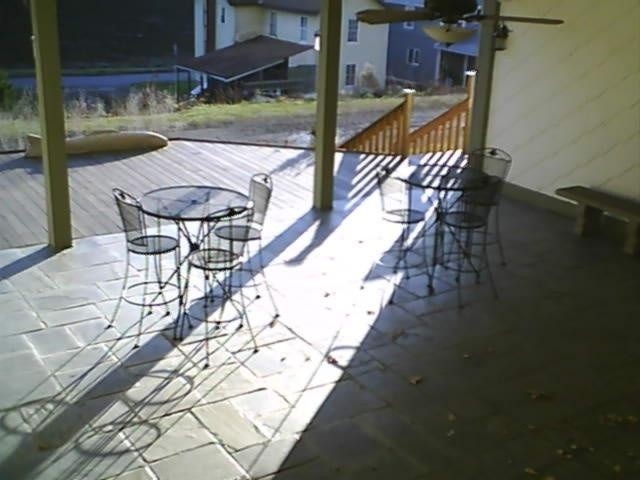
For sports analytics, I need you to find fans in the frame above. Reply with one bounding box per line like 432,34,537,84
354,0,565,49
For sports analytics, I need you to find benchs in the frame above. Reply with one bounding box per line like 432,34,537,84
554,184,639,257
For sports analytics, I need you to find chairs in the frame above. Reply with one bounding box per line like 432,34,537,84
106,172,282,370
359,147,514,308
